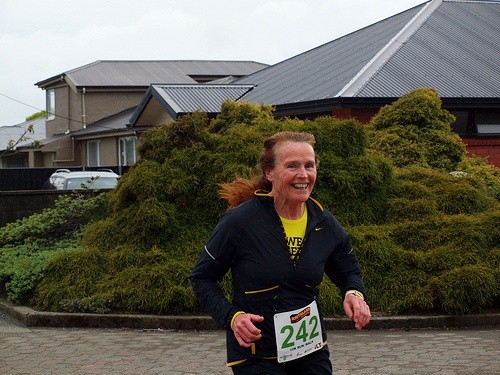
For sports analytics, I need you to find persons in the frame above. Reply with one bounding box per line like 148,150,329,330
192,131,372,375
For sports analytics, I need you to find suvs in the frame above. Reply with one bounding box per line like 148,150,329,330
42,165,121,190
